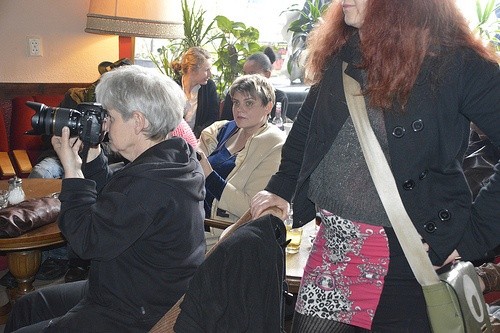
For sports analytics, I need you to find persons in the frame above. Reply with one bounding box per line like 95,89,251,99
189,73,290,234
220,48,288,126
218,0,500,333
87,61,113,104
6,65,205,333
168,49,218,142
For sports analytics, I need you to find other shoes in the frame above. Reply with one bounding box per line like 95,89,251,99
39,256,66,276
65,259,90,282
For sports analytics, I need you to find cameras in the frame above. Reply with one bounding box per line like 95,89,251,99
24,101,109,146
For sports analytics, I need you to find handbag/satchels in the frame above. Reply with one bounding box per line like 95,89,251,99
422,261,492,333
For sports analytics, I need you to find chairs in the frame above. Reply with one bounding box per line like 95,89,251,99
143,203,284,333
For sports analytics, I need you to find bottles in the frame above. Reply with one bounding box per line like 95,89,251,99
273,102,283,129
7,176,24,205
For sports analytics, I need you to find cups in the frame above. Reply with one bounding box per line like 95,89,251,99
283,211,303,254
0,190,8,209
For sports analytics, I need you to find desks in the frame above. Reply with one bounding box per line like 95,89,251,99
0,177,66,305
